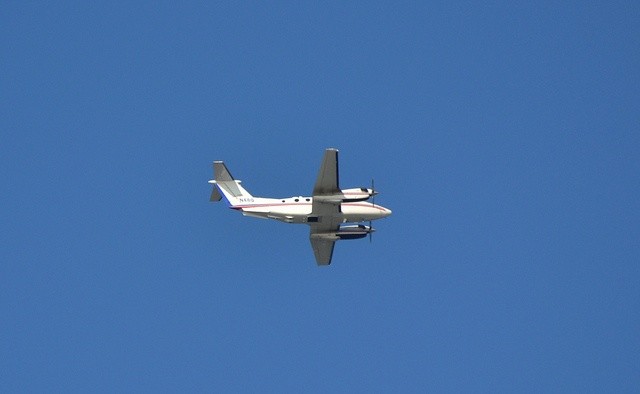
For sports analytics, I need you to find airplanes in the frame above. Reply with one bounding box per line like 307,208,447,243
208,148,393,266
